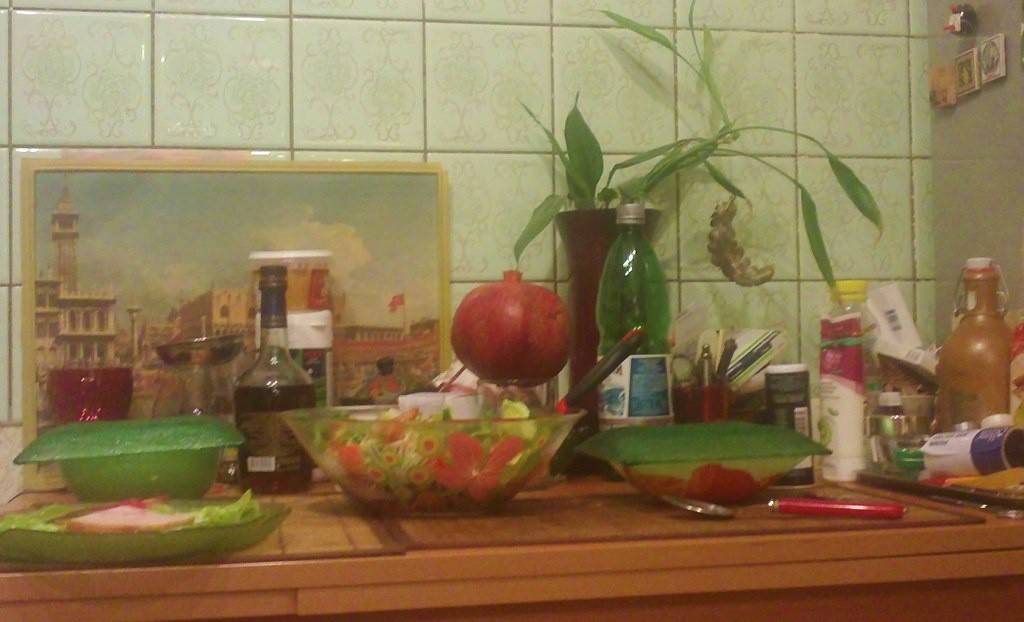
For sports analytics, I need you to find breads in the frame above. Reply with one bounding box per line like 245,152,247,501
67,505,194,533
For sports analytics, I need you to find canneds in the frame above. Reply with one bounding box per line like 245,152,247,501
917,425,1024,479
764,364,815,488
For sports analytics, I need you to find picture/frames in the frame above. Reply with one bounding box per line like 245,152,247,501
20,155,454,493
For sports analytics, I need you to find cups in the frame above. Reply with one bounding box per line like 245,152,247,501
923,428,1024,475
764,365,811,484
903,395,934,436
46,363,132,423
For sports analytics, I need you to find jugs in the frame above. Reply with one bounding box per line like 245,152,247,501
151,333,249,490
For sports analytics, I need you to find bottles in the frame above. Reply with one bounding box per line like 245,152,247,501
599,203,674,432
877,393,903,417
822,279,866,478
234,266,315,492
941,257,1010,425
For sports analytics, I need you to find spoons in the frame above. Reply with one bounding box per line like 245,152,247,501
663,495,908,519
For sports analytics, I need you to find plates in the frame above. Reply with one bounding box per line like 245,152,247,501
0,497,292,564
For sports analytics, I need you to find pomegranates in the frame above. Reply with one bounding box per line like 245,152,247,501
451,270,571,387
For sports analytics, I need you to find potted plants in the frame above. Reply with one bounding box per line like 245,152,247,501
513,1,882,476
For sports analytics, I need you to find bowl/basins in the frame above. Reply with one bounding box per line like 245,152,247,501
279,403,589,511
